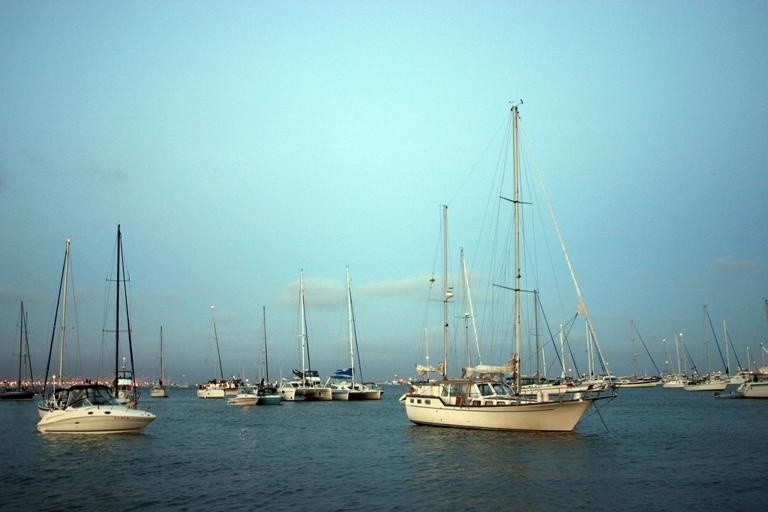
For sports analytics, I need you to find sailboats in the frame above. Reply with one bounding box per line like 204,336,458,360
613,304,768,399
0,222,169,436
197,266,384,408
